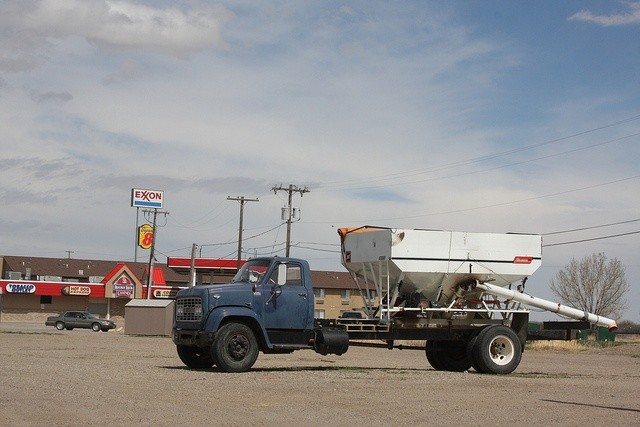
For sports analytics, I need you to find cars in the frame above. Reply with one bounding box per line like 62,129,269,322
45,311,116,332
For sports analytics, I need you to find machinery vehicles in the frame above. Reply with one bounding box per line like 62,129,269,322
172,226,618,375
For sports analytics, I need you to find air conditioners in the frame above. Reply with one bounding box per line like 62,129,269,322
9,272,21,281
